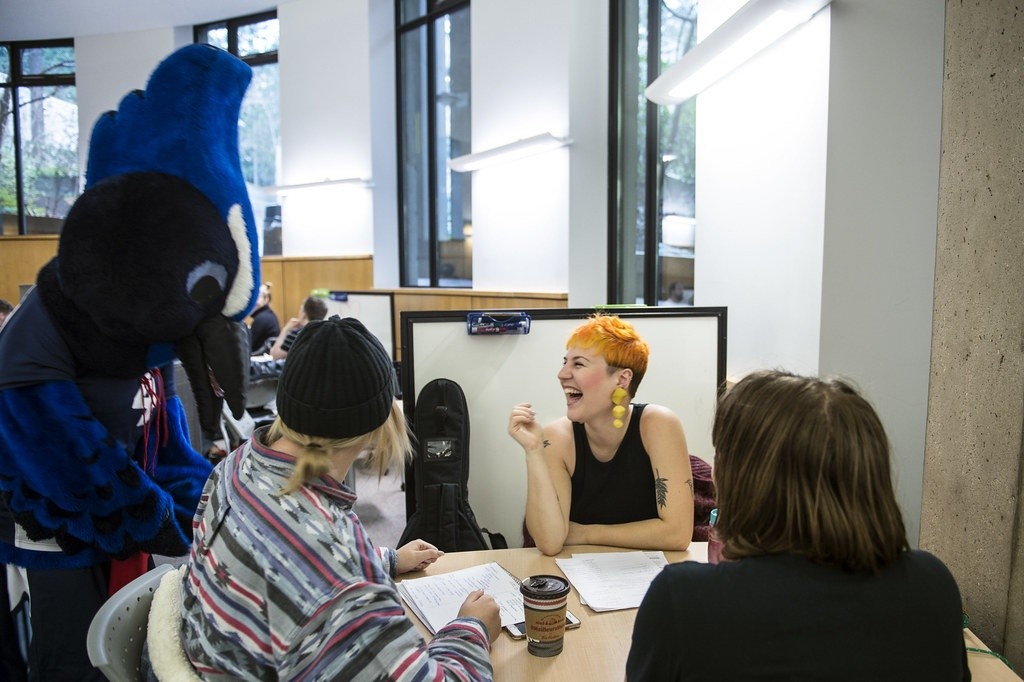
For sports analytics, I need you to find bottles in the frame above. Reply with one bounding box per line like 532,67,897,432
708,509,725,566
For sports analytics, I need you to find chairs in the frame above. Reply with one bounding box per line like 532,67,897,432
85,562,176,682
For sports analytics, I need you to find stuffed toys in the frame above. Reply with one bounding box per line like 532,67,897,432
0,42,263,682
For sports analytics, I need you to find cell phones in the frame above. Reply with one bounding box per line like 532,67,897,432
504,610,581,641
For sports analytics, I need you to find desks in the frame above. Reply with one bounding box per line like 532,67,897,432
388,540,1024,682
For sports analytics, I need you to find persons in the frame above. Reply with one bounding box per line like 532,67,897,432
505,313,694,556
625,370,974,682
144,315,503,681
248,281,281,357
269,297,327,360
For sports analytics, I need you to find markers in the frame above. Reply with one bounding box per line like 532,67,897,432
468,317,527,333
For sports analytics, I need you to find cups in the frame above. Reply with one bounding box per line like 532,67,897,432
521,575,570,657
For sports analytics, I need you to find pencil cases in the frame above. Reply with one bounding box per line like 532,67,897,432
467,312,531,335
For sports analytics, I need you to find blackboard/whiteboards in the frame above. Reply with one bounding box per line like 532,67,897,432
311,290,397,363
400,305,729,551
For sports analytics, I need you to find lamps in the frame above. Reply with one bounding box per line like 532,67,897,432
644,0,841,108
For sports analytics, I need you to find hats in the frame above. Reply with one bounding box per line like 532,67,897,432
277,315,394,440
